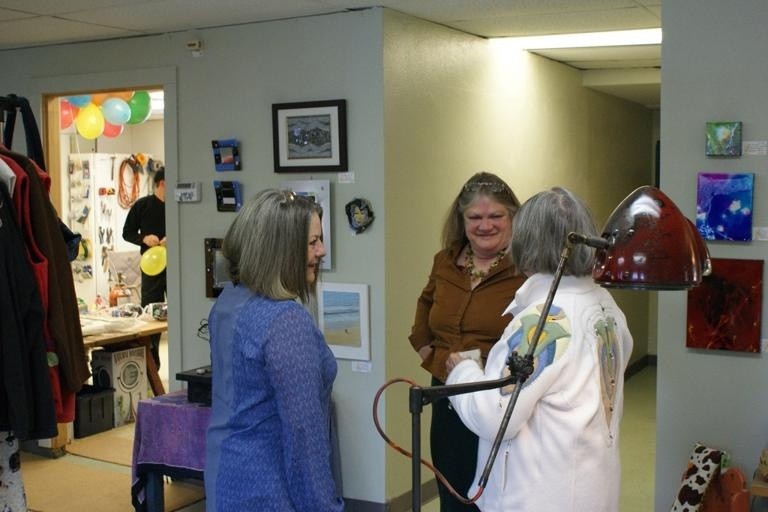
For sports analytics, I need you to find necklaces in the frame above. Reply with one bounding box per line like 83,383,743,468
464,242,509,283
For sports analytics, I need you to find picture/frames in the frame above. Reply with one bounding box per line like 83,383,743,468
204,237,234,299
312,281,372,363
271,99,348,174
211,139,241,173
278,179,332,272
213,180,242,213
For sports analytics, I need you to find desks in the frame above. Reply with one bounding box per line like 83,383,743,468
131,388,214,512
73,312,167,397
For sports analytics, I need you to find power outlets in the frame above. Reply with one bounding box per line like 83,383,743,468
337,173,356,184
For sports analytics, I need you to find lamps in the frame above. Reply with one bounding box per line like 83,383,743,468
371,182,715,512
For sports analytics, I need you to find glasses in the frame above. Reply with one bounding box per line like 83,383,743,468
463,182,510,196
282,188,296,205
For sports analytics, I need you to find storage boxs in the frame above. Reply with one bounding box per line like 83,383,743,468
72,383,117,440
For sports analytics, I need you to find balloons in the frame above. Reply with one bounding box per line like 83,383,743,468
60,90,152,140
140,246,168,277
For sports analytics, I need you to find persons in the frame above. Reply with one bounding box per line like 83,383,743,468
408,171,533,511
122,165,167,372
202,188,348,511
445,186,634,511
350,204,368,226
